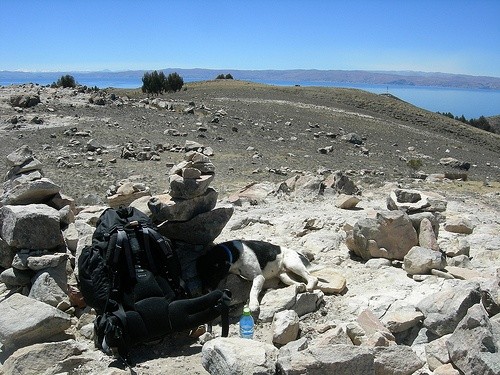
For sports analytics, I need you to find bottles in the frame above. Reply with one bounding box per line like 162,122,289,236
239,308,254,339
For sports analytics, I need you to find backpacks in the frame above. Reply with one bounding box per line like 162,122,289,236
77,206,234,356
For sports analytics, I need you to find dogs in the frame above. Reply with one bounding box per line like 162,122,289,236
196,240,318,312
